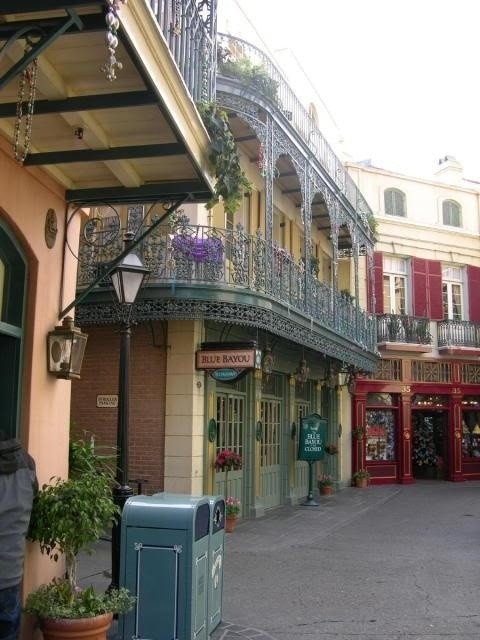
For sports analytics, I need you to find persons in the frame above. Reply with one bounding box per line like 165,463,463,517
0,430,38,640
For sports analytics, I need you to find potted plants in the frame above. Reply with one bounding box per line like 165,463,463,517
351,471,372,487
22,418,138,639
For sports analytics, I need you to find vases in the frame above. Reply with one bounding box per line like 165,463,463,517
437,471,444,481
321,486,332,494
225,515,239,533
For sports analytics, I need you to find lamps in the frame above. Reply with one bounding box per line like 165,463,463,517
47,317,89,379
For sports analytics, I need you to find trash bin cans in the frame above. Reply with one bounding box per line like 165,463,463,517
118,493,226,640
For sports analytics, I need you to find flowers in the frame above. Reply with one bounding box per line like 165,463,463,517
224,497,241,517
324,444,338,457
436,457,445,471
317,472,336,488
214,450,242,500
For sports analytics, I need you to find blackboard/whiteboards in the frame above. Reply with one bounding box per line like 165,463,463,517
297,413,329,461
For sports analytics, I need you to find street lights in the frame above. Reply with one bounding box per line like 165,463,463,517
105,229,153,620
